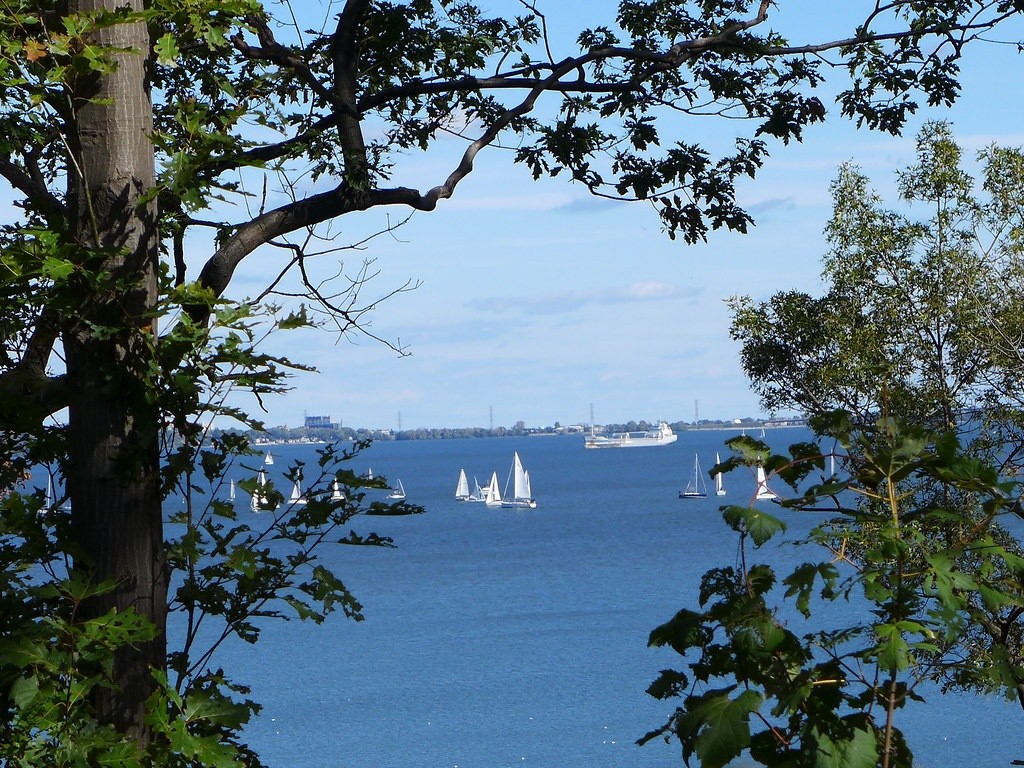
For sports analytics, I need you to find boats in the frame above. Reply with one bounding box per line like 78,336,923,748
582,422,679,450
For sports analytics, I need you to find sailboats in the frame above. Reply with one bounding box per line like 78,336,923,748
249,469,262,513
755,456,777,499
468,477,487,503
456,468,470,503
260,466,280,509
287,470,308,505
227,478,236,502
500,451,537,509
678,453,708,500
715,453,726,496
389,477,406,499
363,468,373,489
35,472,71,521
485,470,504,506
264,450,274,466
329,478,344,500
